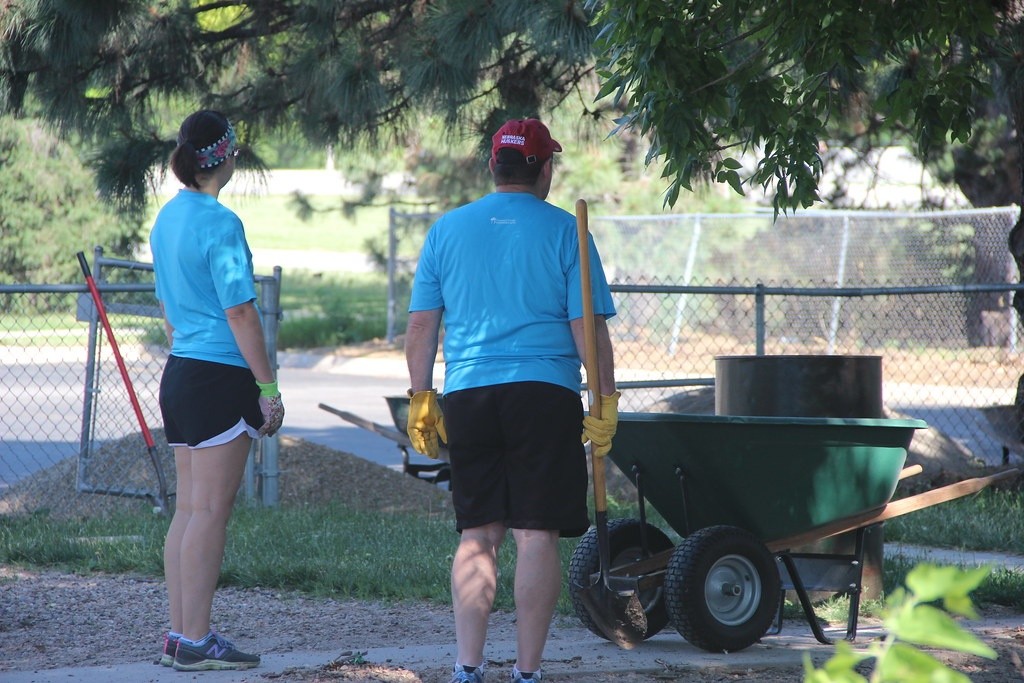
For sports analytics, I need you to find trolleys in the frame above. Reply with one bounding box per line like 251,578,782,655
319,396,448,491
566,410,1023,654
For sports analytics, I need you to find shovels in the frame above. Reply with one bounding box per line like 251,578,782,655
575,198,649,652
77,251,178,519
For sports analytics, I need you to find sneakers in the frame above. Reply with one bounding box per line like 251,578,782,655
159,633,236,667
172,630,260,671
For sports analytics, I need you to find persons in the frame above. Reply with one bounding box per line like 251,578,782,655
406,118,621,683
150,110,285,671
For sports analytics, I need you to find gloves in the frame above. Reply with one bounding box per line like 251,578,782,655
581,391,621,457
407,388,447,459
255,378,284,439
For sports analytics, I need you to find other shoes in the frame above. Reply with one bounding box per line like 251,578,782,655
449,659,485,683
510,663,542,683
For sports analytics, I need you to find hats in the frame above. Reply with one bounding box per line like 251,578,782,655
492,118,562,165
